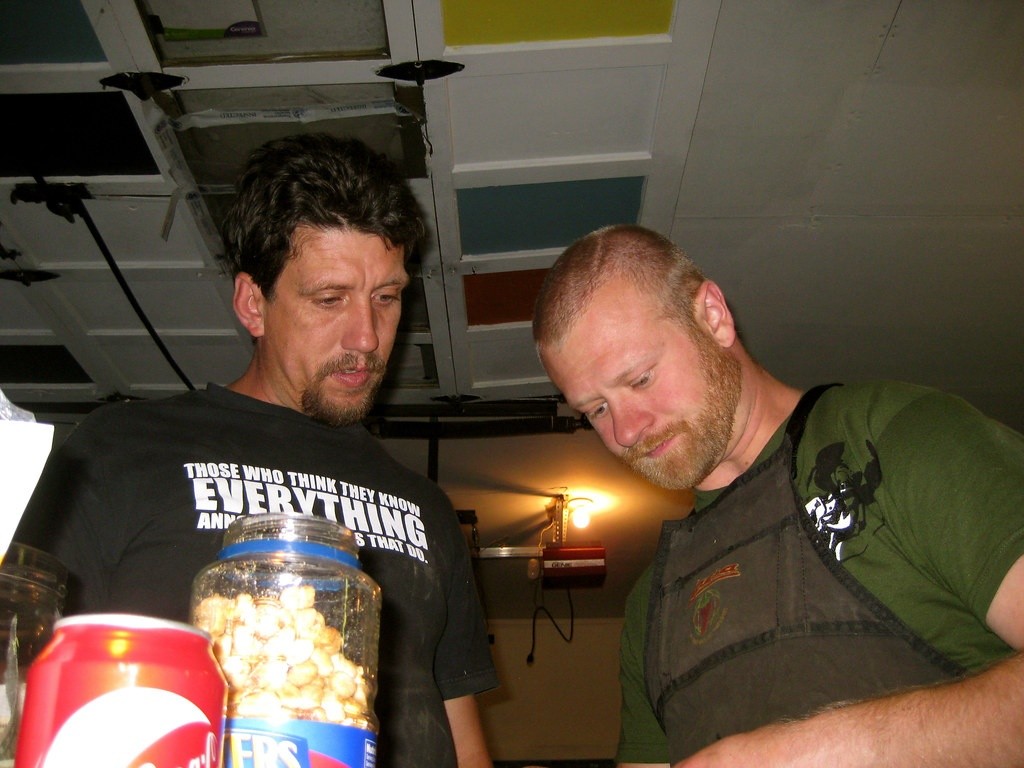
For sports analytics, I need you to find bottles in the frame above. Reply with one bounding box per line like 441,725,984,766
186,512,383,768
0,542,71,768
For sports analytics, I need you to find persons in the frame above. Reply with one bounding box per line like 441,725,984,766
532,225,1024,768
0,134,500,768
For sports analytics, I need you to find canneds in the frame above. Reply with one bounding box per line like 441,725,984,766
10,611,230,768
